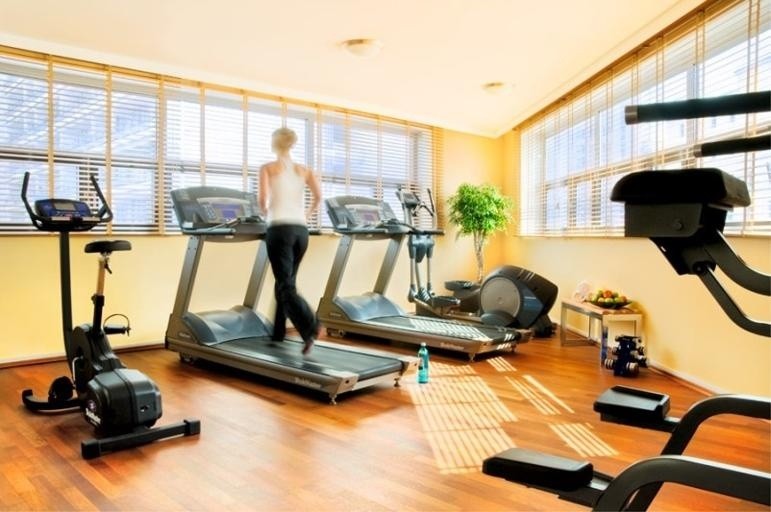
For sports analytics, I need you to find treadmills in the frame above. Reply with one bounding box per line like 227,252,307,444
315,196,532,362
165,186,418,405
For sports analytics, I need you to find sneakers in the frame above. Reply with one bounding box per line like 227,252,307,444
302,324,324,354
271,334,284,342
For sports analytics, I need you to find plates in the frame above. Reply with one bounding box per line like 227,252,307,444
590,301,632,309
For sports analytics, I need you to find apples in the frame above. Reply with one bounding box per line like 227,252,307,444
592,290,628,302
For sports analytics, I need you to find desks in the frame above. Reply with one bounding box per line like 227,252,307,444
560,299,642,366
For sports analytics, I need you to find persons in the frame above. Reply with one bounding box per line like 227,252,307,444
257,127,329,356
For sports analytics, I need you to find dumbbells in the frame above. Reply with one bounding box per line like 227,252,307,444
604,335,646,374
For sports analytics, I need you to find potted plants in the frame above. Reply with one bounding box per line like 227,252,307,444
446,183,516,313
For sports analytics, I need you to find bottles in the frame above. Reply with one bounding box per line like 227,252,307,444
417,341,428,384
